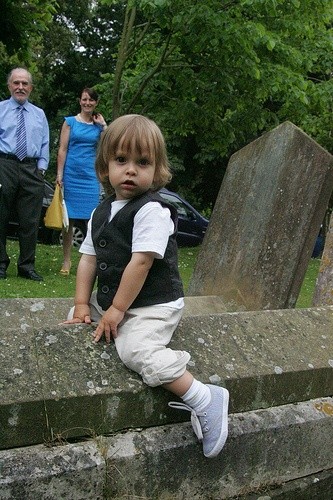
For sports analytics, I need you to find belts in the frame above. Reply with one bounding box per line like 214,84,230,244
0,152,37,162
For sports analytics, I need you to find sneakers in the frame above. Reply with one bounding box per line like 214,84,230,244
167,383,229,458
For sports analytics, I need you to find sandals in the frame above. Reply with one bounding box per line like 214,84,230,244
59,261,72,275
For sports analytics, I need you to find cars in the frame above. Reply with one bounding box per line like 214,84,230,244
6,178,88,247
158,188,210,248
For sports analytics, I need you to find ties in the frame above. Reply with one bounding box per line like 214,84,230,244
15,105,27,161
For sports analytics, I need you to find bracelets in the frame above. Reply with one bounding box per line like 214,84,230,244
103,124,107,127
57,175,63,177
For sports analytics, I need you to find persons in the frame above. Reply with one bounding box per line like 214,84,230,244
63,115,229,459
55,87,107,278
0,68,49,281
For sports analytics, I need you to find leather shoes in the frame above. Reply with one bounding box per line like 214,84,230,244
17,269,43,281
0,270,7,279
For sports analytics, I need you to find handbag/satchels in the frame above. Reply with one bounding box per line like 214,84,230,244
43,182,65,231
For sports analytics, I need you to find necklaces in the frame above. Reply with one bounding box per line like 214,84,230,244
77,113,94,124
